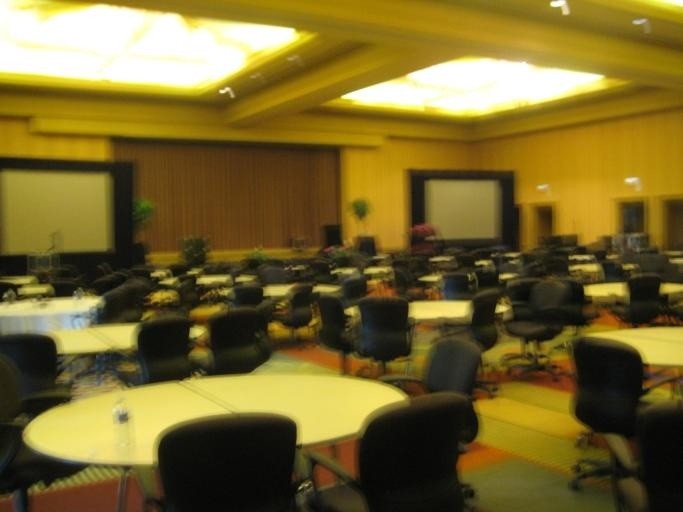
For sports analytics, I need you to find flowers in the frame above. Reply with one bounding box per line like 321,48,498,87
408,223,437,240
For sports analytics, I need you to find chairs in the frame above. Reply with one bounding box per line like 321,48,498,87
0,223,683,511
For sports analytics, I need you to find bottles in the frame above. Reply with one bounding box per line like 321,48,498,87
114,401,133,446
